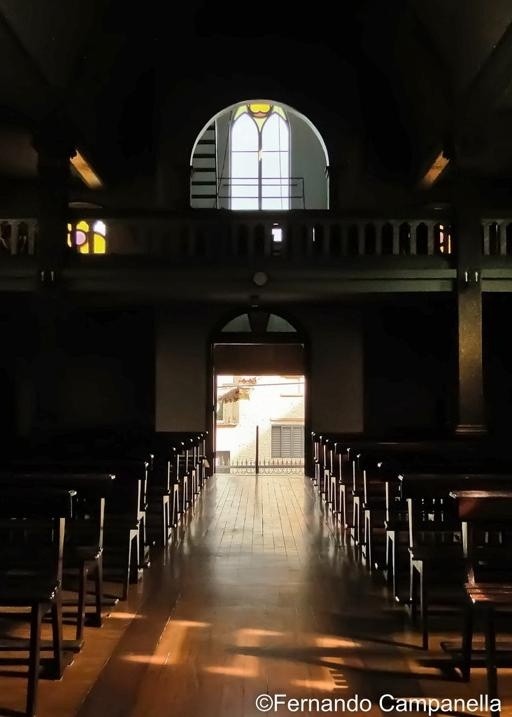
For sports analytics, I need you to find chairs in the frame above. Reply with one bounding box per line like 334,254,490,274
0,428,207,478
0,484,78,710
379,460,512,602
356,449,512,575
0,486,107,655
0,477,206,580
443,488,512,712
308,430,356,537
399,473,512,650
0,471,139,604
